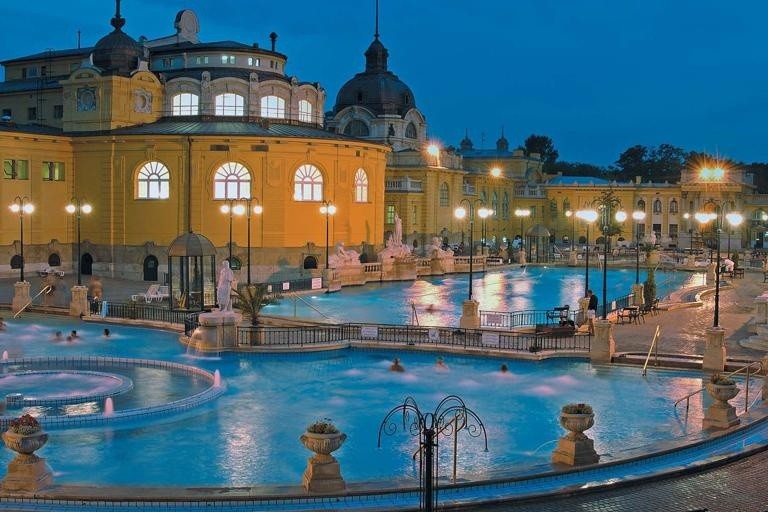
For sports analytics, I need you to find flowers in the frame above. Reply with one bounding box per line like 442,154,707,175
710,372,736,385
306,415,338,434
9,413,42,435
560,402,594,415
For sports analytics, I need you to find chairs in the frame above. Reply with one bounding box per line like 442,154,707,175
716,265,768,283
616,294,663,325
147,285,168,303
132,284,160,304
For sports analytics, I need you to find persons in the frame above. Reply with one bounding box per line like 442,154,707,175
559,305,575,332
394,211,402,246
101,329,111,338
432,357,448,372
0,322,5,330
71,331,80,340
505,241,512,265
390,358,404,372
62,336,74,345
587,290,598,335
217,260,233,312
51,331,63,341
337,242,345,258
720,258,735,272
0,317,6,327
498,364,511,376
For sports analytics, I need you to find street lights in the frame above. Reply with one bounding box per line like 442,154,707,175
455,196,487,297
696,198,743,328
631,208,646,282
316,200,337,267
240,197,263,286
514,207,531,247
220,194,243,264
724,212,731,259
67,197,92,284
588,192,628,320
564,209,582,252
582,208,597,298
477,209,497,254
683,212,699,256
10,192,35,280
